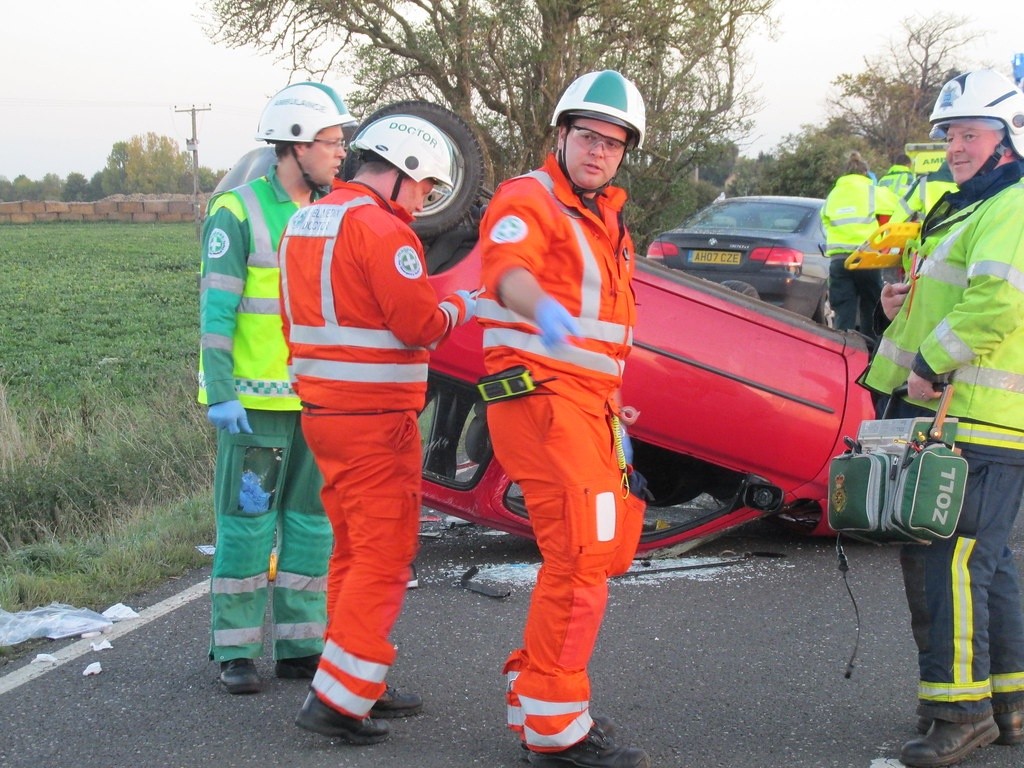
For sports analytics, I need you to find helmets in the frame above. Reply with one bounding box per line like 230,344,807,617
929,69,1024,159
255,83,358,144
349,114,454,189
550,70,646,151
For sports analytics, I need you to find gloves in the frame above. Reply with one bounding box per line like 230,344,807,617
208,400,253,434
534,296,580,346
455,290,476,325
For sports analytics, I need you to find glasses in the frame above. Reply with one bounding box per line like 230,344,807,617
315,139,345,150
567,124,628,157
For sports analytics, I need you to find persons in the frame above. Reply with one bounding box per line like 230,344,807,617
857,67,1024,768
194,82,358,694
822,152,960,331
278,113,477,745
479,68,648,768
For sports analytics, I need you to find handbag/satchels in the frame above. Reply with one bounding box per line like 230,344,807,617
827,381,969,546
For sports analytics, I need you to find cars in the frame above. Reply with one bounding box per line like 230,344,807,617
212,100,889,571
650,195,854,329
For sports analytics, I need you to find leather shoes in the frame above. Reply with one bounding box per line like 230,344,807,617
370,684,424,719
294,690,391,745
527,725,651,768
275,653,322,680
916,708,1024,746
219,658,260,693
521,715,618,760
899,713,1000,767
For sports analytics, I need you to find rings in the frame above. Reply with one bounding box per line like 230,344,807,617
921,393,926,398
